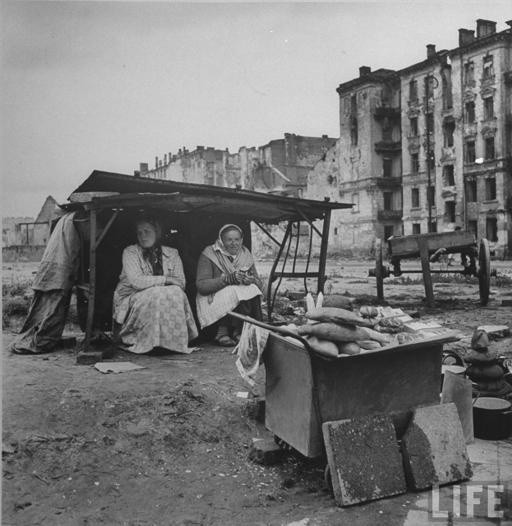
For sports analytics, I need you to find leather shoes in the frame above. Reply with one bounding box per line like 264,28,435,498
213,333,241,347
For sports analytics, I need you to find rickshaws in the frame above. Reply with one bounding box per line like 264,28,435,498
369,225,493,312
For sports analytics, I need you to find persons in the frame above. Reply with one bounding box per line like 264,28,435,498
110,215,201,355
193,221,264,347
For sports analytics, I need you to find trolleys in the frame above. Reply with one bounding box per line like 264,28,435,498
229,306,461,497
